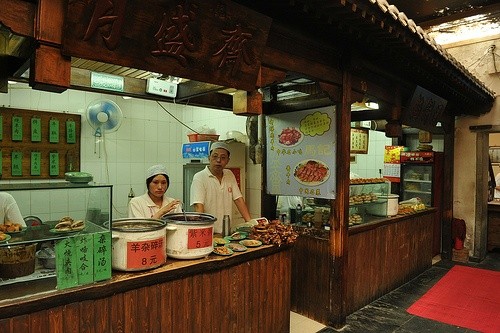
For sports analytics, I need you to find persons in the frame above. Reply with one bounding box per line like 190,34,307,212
128,165,183,219
494,172,500,198
0,193,27,228
189,142,252,235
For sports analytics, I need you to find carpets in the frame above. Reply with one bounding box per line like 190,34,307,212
407,265,500,333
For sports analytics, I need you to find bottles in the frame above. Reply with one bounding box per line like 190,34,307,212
296,204,303,215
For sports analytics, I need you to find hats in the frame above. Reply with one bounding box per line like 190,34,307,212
209,142,231,156
145,166,169,180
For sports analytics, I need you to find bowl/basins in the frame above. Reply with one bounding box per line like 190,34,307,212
35,250,56,269
0,243,38,280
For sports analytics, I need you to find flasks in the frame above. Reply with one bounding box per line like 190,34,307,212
223,214,230,237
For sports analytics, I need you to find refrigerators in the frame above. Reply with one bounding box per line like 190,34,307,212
182,141,247,231
400,151,445,211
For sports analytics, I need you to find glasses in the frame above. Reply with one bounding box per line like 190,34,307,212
210,154,228,160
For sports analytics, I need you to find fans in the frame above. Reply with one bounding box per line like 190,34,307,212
86,97,124,138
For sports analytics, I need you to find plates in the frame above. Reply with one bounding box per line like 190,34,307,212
213,224,268,256
48,226,87,234
6,227,27,234
65,171,94,185
293,159,331,187
23,216,41,226
0,234,11,242
277,127,305,147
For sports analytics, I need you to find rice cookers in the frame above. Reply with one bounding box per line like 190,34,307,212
365,193,400,218
102,216,177,272
160,212,218,260
